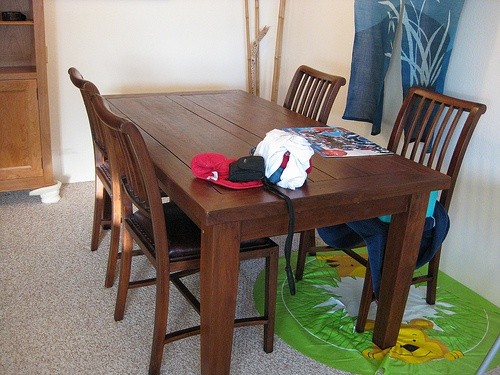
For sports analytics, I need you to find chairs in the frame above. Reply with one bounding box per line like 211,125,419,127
274,66,346,256
89,93,279,375
296,84,487,333
67,66,223,289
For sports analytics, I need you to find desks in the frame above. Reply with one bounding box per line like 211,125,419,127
103,89,451,375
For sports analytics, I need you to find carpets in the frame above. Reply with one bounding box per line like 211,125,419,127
253,244,500,375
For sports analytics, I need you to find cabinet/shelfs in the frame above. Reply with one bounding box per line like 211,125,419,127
0,0,58,195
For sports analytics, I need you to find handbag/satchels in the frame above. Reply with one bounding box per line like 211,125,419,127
229,156,265,181
